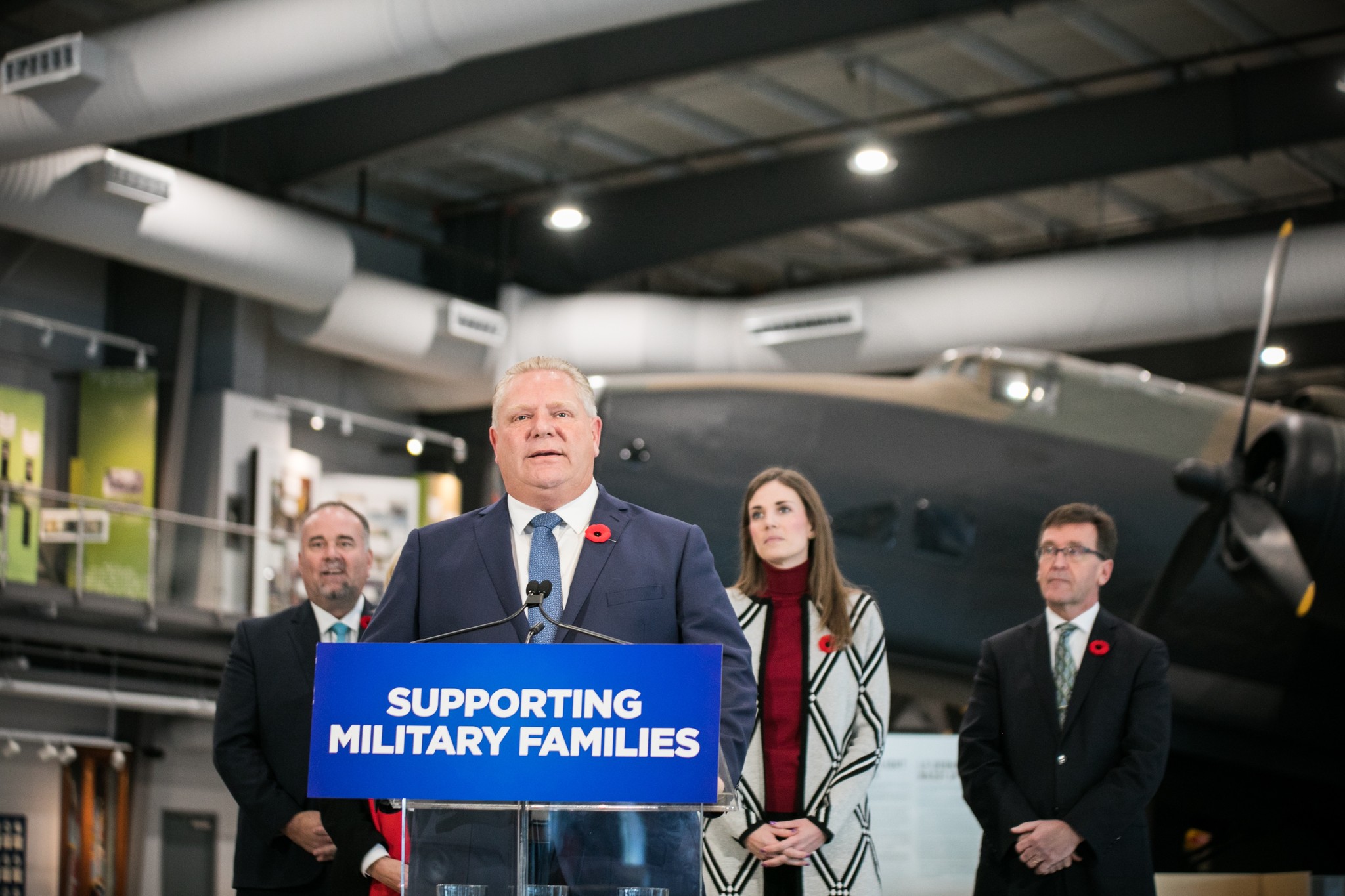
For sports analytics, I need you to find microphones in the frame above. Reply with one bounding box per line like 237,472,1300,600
409,580,636,644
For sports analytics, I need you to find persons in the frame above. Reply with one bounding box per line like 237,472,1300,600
212,501,379,896
319,795,413,896
958,502,1177,896
694,468,890,896
362,354,759,896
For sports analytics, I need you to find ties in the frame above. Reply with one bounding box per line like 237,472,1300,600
329,622,351,643
527,513,564,643
1054,623,1080,727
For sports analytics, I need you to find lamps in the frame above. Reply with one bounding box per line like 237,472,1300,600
535,127,594,242
840,56,903,180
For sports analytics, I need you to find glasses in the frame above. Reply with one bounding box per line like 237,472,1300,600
1034,544,1106,561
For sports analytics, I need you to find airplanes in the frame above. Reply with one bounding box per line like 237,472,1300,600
565,213,1344,875
